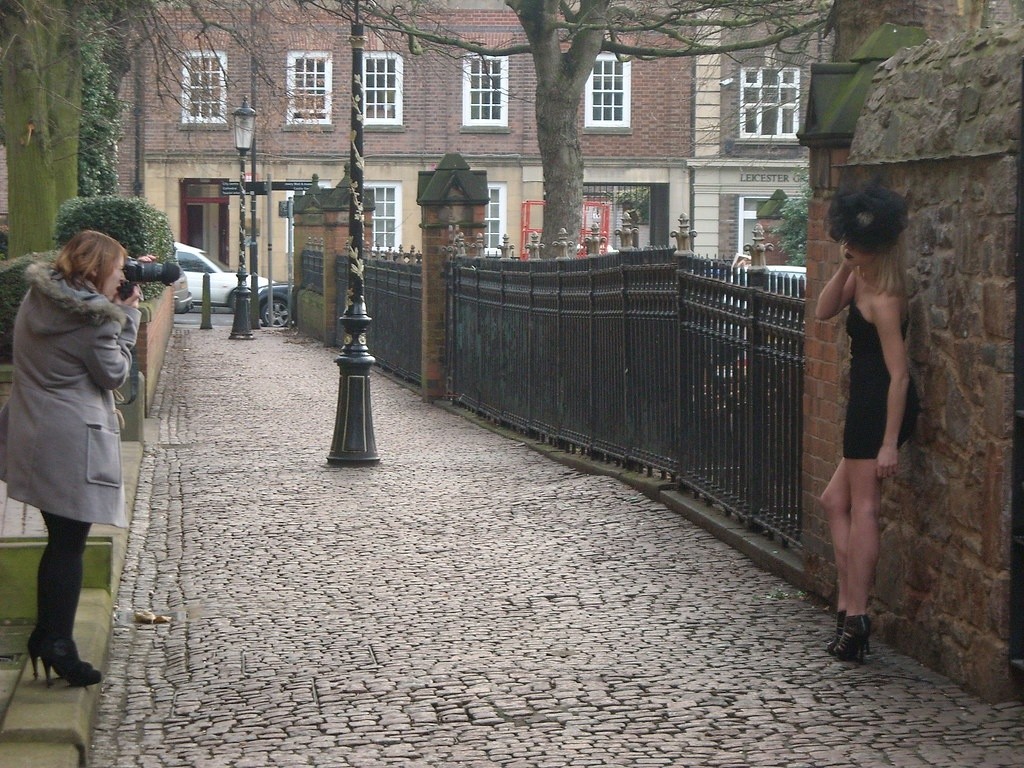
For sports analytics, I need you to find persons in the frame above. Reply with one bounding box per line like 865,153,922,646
815,181,920,662
0,230,157,690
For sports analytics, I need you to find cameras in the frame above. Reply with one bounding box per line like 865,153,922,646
118,256,181,301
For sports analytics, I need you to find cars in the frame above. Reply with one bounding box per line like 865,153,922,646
173,264,194,314
258,283,292,328
174,240,277,307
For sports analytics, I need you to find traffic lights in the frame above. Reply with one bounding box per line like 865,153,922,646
229,94,254,340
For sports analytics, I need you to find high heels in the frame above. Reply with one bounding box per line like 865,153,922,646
27,626,92,679
831,614,869,664
826,610,870,654
40,638,103,687
